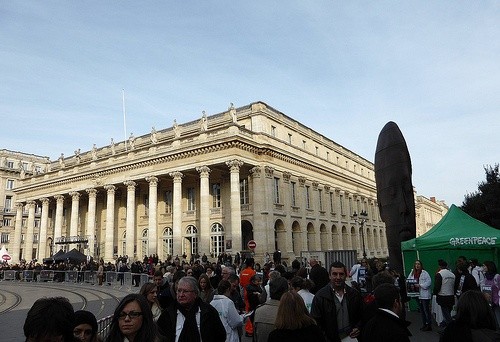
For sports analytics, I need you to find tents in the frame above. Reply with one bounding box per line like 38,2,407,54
402,204,500,313
43,248,92,264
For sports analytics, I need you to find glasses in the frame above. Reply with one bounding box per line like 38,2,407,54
188,272,193,274
176,289,197,293
117,311,142,319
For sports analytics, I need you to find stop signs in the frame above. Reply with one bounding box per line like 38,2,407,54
247,241,256,250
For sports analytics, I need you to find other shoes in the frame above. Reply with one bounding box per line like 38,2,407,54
134,285,139,287
245,333,252,337
420,327,432,331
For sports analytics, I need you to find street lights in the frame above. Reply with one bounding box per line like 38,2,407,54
352,209,369,259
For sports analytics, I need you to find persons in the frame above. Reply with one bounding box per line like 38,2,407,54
268,291,324,342
254,277,288,342
209,281,245,342
24,296,82,342
72,310,109,342
106,294,165,342
115,249,331,337
348,256,408,319
407,260,432,331
311,262,362,342
358,284,411,342
157,275,227,342
433,256,500,328
439,290,500,342
0,258,115,286
